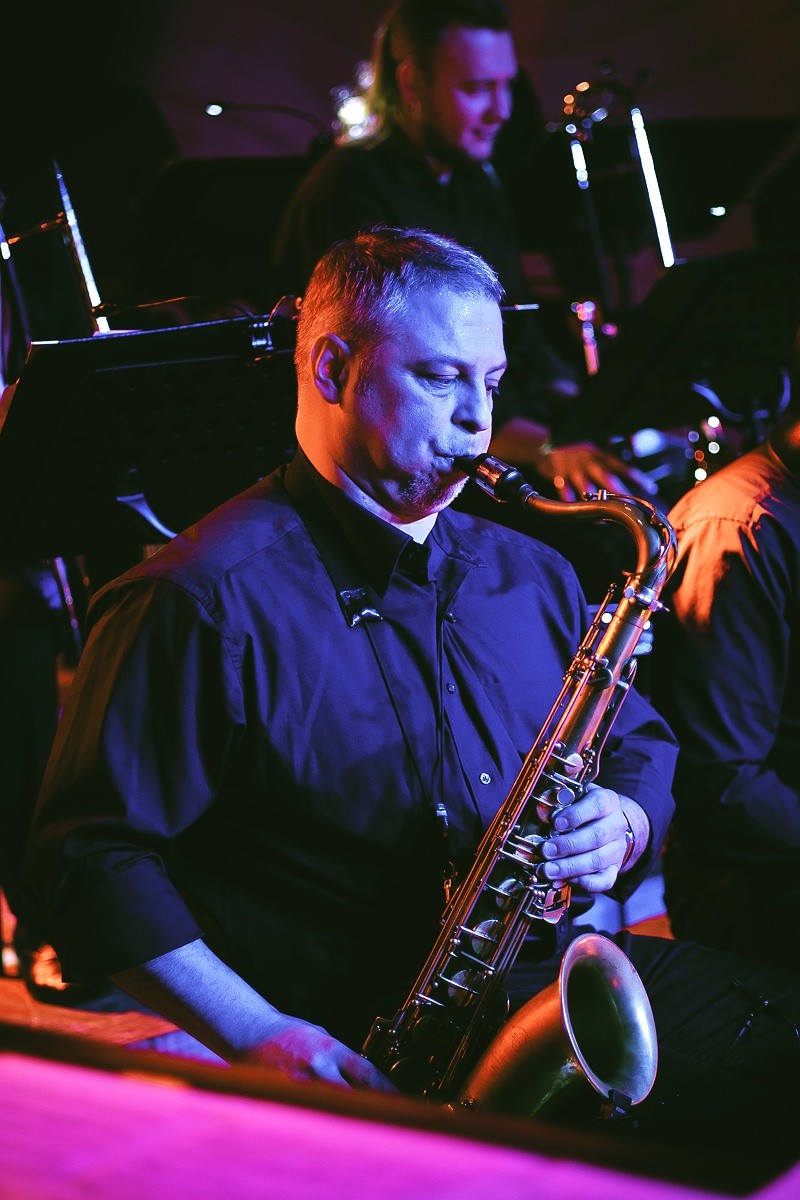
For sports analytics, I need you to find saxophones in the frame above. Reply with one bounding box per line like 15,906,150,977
357,452,679,1120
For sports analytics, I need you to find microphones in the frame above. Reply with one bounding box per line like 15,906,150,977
88,292,261,321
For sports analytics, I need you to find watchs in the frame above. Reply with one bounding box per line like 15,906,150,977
621,811,636,881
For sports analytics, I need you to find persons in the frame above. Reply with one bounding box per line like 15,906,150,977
0,231,141,1014
615,413,800,1013
240,0,660,500
30,225,800,1140
